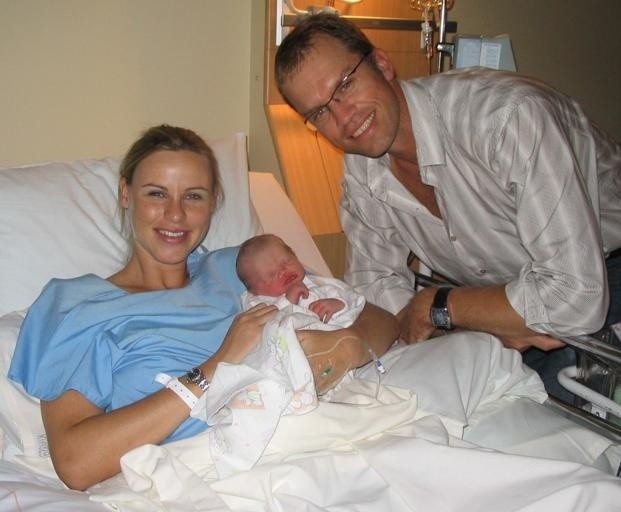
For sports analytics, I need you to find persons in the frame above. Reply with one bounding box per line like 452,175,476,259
273,13,620,408
238,235,361,324
6,124,403,491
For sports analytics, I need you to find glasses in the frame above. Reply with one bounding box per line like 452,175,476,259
304,63,359,133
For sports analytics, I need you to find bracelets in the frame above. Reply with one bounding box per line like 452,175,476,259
156,372,200,414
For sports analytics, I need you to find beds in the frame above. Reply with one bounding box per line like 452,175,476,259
1,169,621,510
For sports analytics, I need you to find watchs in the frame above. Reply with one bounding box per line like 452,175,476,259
431,286,456,333
187,364,211,393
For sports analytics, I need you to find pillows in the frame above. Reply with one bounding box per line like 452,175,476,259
1,130,251,317
